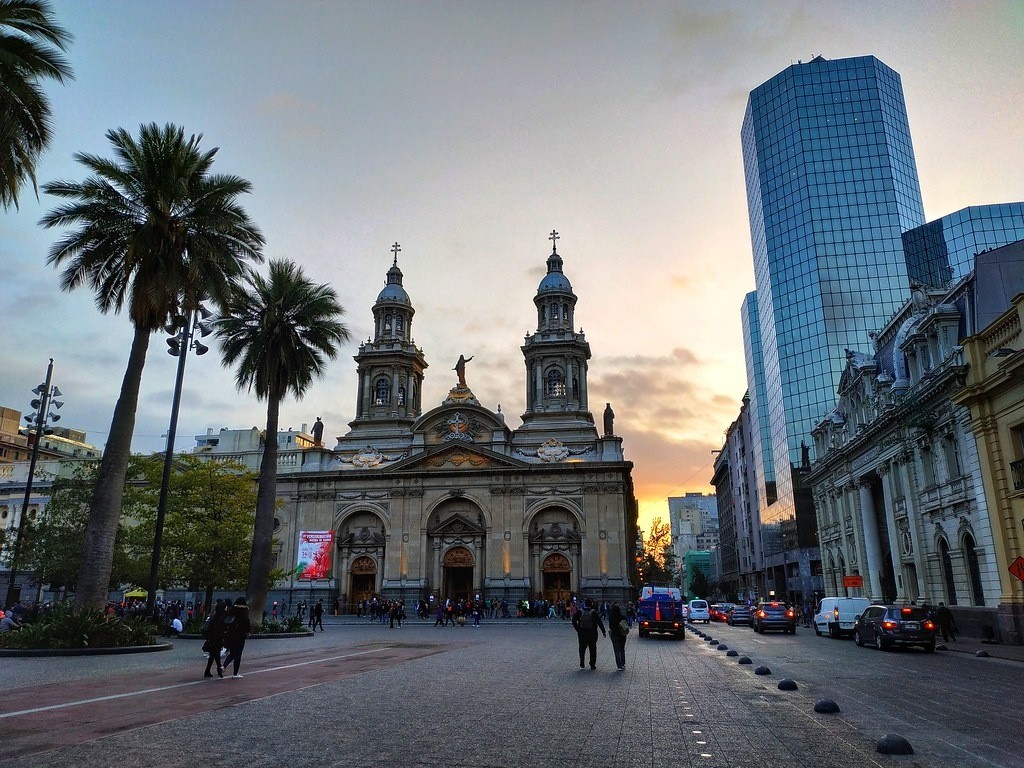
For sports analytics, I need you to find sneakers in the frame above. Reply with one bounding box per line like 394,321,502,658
220,665,226,672
232,674,244,680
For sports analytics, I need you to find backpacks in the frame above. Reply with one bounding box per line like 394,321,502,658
222,616,238,639
579,607,595,630
199,615,217,636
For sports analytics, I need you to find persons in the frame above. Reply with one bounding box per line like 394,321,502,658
220,597,250,678
310,417,324,446
571,599,607,671
203,602,225,678
602,402,615,436
788,602,819,627
0,585,641,638
451,354,474,387
608,604,629,670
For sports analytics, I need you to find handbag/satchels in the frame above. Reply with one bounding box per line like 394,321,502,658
496,601,500,608
952,626,960,634
618,619,630,637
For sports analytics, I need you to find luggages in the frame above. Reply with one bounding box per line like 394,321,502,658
453,612,466,627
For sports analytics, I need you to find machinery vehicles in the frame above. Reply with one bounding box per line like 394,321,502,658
636,587,685,639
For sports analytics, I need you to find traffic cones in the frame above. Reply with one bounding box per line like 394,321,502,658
655,601,661,621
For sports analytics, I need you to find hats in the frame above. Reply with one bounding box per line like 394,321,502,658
215,602,228,608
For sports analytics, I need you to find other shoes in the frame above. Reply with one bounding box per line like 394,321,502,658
321,629,324,631
590,665,595,670
580,665,584,668
618,664,625,671
472,625,480,628
312,630,316,632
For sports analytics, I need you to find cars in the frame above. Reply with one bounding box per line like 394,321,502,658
710,601,753,628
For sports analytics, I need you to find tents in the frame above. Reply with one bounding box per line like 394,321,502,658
122,586,149,606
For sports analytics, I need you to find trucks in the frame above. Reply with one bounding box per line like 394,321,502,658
813,597,870,639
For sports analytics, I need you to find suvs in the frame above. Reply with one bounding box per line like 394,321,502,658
752,602,795,634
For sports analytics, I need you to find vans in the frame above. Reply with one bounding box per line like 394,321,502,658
685,600,710,624
855,605,935,654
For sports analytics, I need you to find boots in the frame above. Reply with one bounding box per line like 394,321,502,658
204,658,213,678
215,657,223,678
